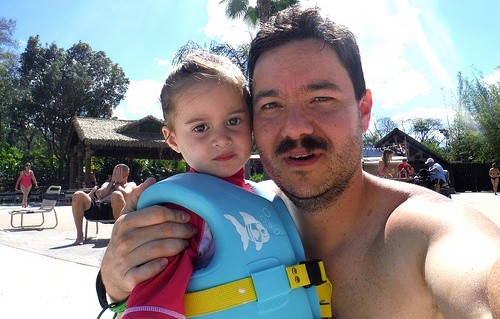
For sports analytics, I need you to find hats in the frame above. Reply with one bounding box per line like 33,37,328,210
425,158,434,164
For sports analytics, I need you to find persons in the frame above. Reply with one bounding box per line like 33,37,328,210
95,4,500,319
119,50,335,319
375,149,394,179
488,162,500,195
93,168,101,182
72,164,136,245
397,158,413,180
424,157,447,193
396,143,406,157
14,162,39,208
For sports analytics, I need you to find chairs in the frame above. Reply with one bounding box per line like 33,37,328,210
83,218,115,243
8,185,61,232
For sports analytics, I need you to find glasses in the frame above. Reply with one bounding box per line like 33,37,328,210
25,164,30,166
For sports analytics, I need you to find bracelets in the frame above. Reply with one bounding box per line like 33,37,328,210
95,267,132,319
115,181,122,186
